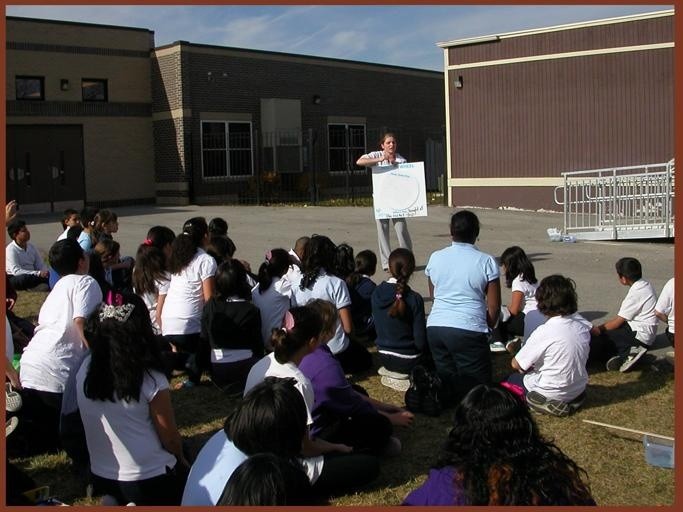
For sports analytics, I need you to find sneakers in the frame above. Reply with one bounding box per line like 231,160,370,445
526,391,570,417
6,416,25,441
665,351,675,366
569,390,587,409
505,337,521,358
27,283,49,292
421,371,442,417
380,376,411,392
379,436,402,458
606,355,624,371
378,366,409,380
619,345,648,373
223,381,246,400
489,341,507,352
6,382,24,414
405,365,433,413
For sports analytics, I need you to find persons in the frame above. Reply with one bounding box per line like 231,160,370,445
6,200,595,505
590,257,659,372
655,278,675,347
356,131,416,272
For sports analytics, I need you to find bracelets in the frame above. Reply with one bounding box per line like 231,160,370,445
598,323,607,333
